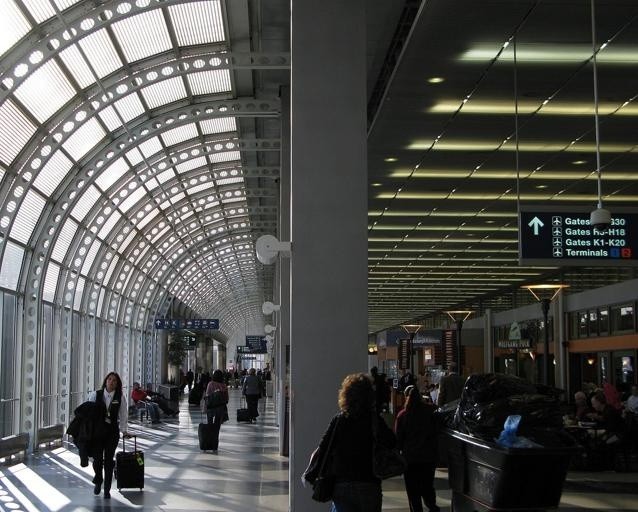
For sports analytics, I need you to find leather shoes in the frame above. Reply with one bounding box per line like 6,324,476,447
94,485,110,498
249,416,256,421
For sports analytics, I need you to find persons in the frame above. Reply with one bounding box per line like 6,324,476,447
178,366,270,426
131,382,181,424
369,366,446,512
301,373,398,512
88,372,131,499
564,382,638,471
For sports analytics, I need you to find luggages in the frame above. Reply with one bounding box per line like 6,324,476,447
198,412,219,450
116,435,144,488
237,397,250,421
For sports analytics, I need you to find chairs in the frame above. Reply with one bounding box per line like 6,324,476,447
608,415,635,472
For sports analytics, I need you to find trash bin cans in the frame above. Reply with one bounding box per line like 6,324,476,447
158,384,179,414
266,380,273,397
441,427,585,512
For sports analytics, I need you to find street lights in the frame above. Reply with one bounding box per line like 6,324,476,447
396,324,424,378
438,309,478,375
519,282,570,386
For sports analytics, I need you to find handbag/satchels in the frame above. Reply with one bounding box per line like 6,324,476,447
311,469,332,503
203,390,228,410
